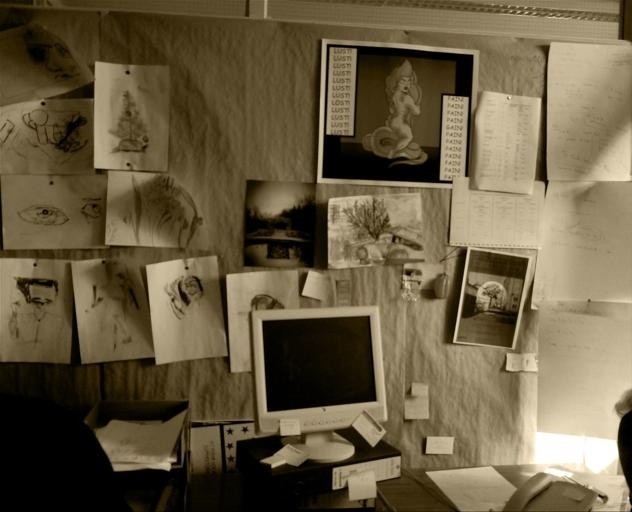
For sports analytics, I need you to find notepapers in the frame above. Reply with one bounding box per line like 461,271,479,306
259,454,286,469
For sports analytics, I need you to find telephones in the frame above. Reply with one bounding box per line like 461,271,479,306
502,471,599,512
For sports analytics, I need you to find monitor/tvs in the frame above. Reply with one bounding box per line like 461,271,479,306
249,305,388,463
